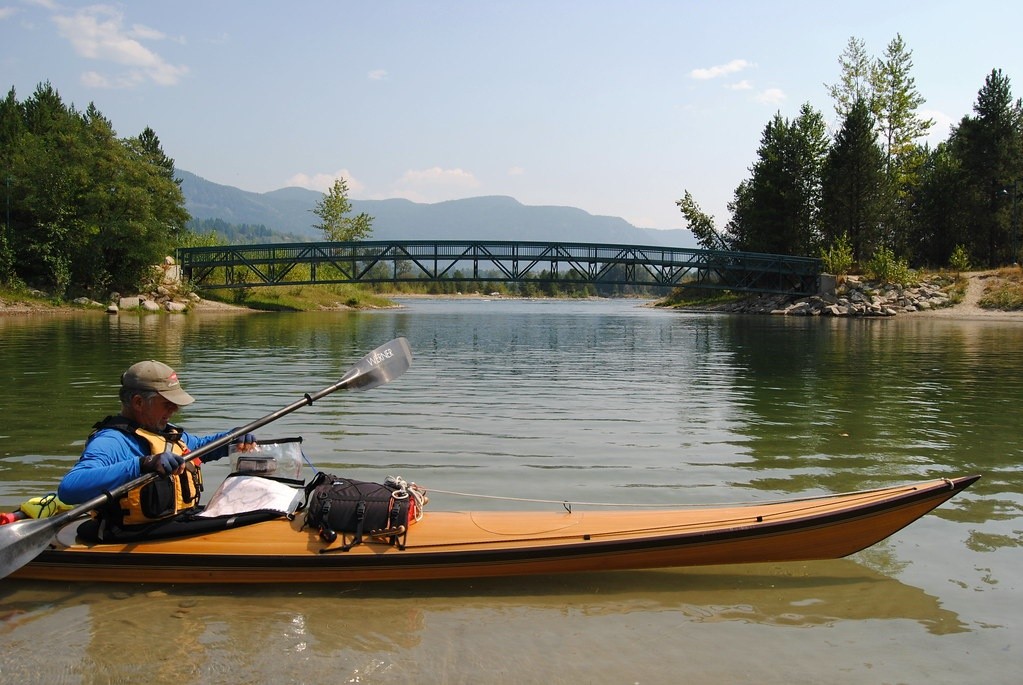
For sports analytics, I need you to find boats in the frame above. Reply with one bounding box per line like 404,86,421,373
2,473,984,583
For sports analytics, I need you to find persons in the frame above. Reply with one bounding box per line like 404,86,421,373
58,360,257,529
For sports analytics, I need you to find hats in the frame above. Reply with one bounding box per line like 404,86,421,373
121,360,195,406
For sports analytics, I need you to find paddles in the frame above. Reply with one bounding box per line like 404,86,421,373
0,335,413,582
